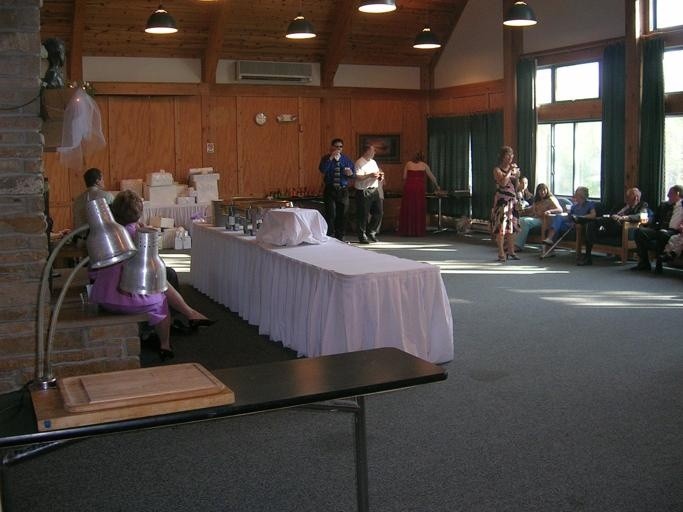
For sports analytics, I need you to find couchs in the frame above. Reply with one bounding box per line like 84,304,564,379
579,209,654,267
544,201,607,264
512,196,573,258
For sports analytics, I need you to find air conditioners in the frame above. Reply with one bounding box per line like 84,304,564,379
235,59,315,86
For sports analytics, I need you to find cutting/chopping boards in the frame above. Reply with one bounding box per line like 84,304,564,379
28,380,236,432
56,363,224,413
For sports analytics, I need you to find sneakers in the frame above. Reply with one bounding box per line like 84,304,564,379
571,215,580,224
540,251,557,258
542,239,554,246
578,256,594,266
368,232,378,242
358,235,370,244
654,263,664,274
631,262,652,271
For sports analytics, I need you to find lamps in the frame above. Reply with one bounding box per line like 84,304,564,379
144,5,178,34
35,228,170,390
285,13,318,40
414,29,440,50
358,1,398,15
503,3,537,27
33,197,138,390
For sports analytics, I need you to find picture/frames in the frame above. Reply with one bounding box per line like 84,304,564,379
356,132,401,165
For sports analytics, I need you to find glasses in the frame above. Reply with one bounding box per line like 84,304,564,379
333,145,343,148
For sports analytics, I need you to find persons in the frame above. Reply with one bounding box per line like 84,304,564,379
540,187,596,259
88,188,216,362
517,176,533,208
575,187,649,267
397,151,440,238
72,167,114,245
490,145,521,261
513,184,564,253
629,185,683,275
352,143,385,245
140,263,197,347
318,138,356,241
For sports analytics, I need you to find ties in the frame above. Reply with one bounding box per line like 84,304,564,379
332,160,341,189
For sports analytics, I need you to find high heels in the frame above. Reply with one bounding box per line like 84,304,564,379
506,252,520,261
160,346,174,361
188,318,219,331
498,254,506,261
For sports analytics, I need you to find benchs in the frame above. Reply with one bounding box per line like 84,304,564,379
50,240,86,259
49,264,148,378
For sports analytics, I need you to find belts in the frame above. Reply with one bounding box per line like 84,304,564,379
76,235,87,241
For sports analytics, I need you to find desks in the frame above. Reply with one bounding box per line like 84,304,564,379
1,345,448,512
143,199,208,249
189,217,454,364
290,190,473,235
